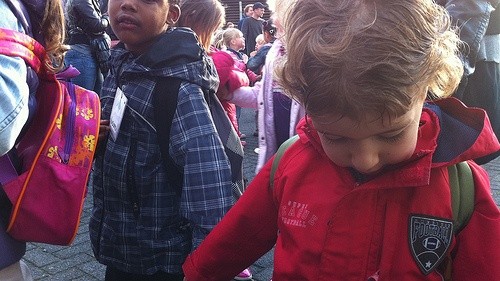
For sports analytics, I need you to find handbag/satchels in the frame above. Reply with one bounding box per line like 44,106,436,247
87,29,112,74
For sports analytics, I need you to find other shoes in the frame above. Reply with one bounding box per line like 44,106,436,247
235,267,253,280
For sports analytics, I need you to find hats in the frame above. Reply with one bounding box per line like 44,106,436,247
253,1,268,8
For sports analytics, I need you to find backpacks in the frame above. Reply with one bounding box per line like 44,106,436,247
0,27,100,248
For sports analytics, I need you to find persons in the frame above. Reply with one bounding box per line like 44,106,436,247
0,1,70,280
182,0,500,281
52,0,110,92
432,0,499,166
174,0,309,280
87,0,234,281
